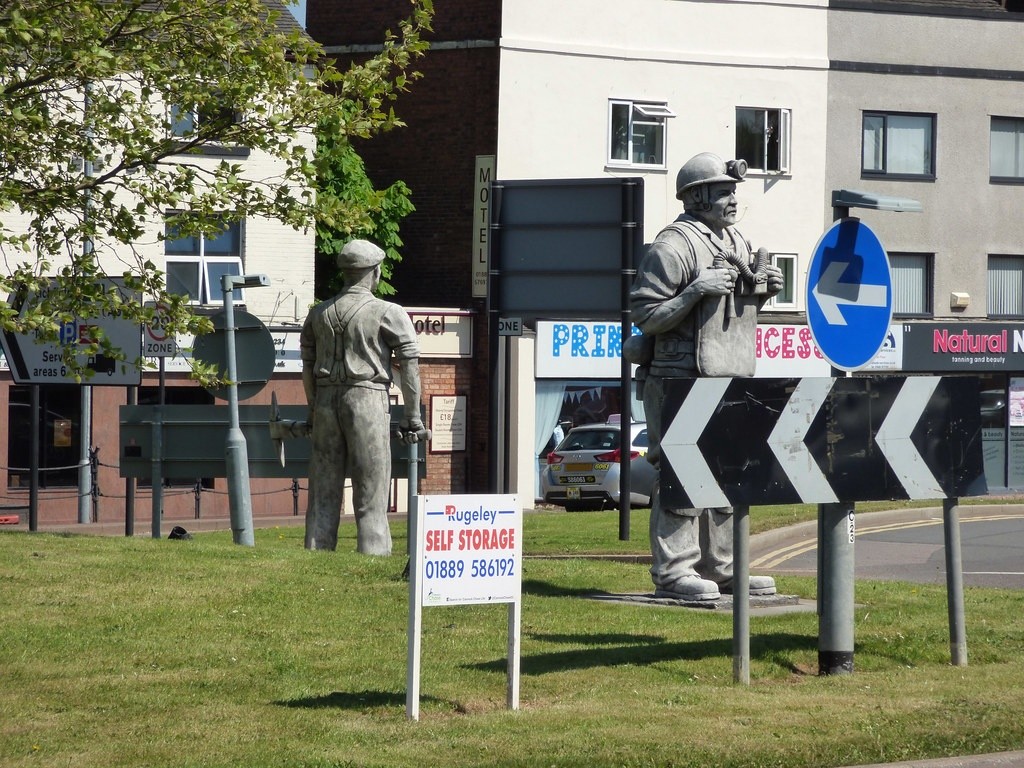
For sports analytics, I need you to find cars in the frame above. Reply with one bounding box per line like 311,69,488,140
980,390,1005,417
541,414,659,508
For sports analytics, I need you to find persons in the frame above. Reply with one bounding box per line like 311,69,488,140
625,153,779,603
297,240,426,556
